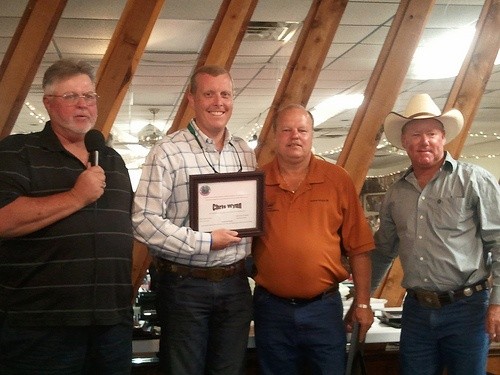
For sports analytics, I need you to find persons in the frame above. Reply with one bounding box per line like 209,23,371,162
253,104,374,375
344,94,500,375
132,65,257,375
0,58,136,375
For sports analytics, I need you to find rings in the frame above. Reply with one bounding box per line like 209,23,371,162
102,182,106,188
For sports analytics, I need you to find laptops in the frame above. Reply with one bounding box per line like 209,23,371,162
382,310,403,323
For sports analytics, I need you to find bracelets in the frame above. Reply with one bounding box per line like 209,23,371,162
354,303,372,308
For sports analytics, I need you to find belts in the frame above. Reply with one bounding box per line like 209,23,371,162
157,258,246,281
274,288,337,308
407,279,487,309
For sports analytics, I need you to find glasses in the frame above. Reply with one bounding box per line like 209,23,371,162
44,92,100,106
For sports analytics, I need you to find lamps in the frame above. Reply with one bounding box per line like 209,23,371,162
140,107,165,147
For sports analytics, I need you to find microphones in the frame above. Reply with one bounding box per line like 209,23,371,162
84,129,104,165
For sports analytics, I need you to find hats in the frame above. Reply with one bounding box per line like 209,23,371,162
383,93,464,150
